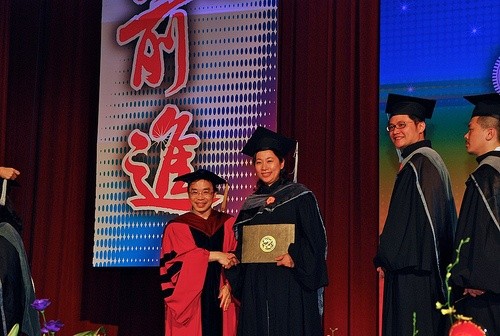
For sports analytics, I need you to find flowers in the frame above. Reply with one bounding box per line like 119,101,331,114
30,299,64,336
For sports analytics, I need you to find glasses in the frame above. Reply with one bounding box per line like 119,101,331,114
189,191,214,197
386,121,415,132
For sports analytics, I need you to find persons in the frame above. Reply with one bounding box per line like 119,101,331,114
0,167,20,180
221,125,330,336
158,169,241,336
432,92,500,336
372,92,459,336
0,177,42,336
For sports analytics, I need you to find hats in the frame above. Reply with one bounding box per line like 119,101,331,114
385,93,436,121
463,93,500,119
172,169,226,185
240,126,298,183
